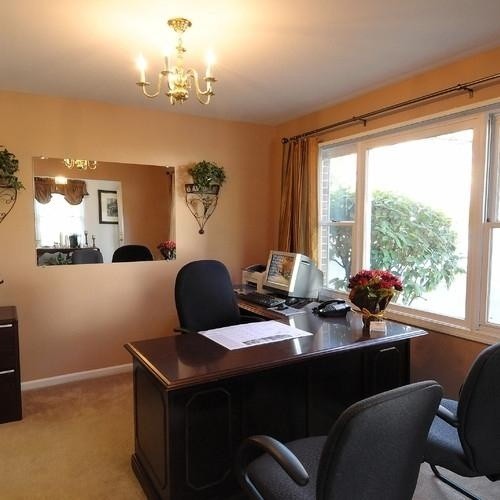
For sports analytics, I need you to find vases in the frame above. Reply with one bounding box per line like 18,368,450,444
361,310,385,329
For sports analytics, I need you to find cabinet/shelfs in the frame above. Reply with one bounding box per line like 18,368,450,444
0,301,25,424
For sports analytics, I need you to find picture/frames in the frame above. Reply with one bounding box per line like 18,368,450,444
97,188,119,225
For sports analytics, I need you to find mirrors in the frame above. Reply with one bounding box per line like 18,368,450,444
30,153,178,268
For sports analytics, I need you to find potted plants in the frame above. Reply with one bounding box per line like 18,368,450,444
1,144,29,193
189,159,228,192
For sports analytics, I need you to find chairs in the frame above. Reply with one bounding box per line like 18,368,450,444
72,246,105,265
235,373,445,498
112,244,154,264
170,257,257,337
421,336,499,499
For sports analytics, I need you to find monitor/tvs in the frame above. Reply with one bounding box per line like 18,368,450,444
262,249,323,306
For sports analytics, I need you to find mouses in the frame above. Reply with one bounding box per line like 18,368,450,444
240,291,286,308
277,304,288,310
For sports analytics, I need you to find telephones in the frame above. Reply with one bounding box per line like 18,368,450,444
317,298,351,317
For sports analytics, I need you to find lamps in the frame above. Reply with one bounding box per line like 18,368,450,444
130,15,217,109
54,177,67,185
61,158,100,172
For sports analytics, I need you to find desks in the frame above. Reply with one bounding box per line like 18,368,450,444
117,309,429,500
36,246,98,264
235,282,322,318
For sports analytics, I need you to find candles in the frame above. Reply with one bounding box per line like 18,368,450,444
83,230,88,236
91,234,96,239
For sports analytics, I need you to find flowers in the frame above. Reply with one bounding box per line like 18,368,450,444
347,267,405,314
155,240,176,260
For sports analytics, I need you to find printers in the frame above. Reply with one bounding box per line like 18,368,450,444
241,263,274,295
